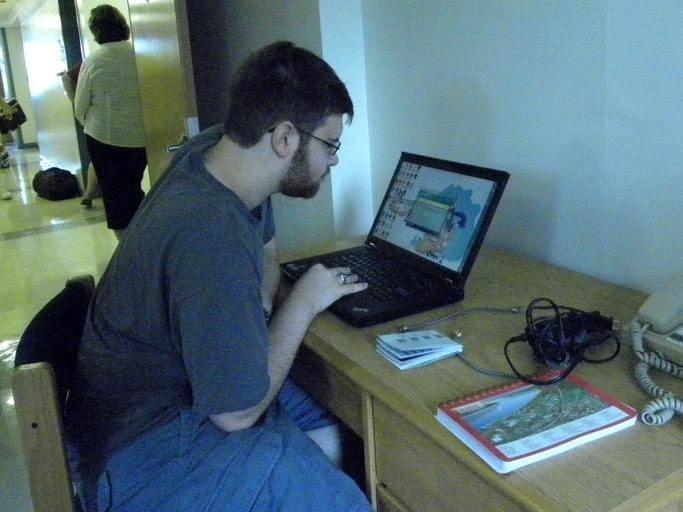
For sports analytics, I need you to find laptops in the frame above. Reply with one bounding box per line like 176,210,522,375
280,151,510,328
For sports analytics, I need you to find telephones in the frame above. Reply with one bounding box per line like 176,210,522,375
621,272,683,366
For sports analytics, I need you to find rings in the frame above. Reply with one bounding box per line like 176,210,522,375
336,272,348,285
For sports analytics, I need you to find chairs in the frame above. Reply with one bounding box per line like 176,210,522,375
11,274,96,511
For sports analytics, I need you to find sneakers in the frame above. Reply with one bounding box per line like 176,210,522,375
80,198,91,207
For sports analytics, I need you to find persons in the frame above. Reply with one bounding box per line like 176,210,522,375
70,4,149,244
59,38,383,511
0,71,15,201
59,60,106,208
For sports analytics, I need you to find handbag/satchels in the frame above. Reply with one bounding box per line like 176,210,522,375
1,99,27,134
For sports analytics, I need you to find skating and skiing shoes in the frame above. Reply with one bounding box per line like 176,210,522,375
1,188,12,199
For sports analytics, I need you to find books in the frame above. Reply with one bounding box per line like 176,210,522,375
433,367,640,475
374,328,463,370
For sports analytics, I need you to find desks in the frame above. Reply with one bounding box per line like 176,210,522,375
260,237,682,512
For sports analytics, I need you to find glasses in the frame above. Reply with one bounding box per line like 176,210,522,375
268,123,342,156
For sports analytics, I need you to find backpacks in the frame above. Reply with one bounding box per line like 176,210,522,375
32,167,82,199
